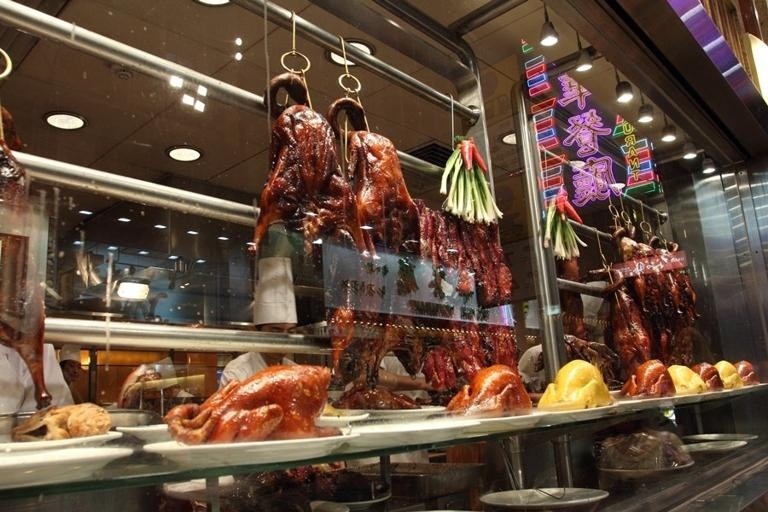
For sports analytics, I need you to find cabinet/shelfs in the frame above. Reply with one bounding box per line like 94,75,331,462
3,384,768,512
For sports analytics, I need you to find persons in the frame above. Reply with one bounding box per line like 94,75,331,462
358,318,438,465
153,357,194,415
58,343,85,405
0,328,74,417
515,339,547,403
215,322,300,395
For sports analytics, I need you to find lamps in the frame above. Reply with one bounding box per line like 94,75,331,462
614,67,642,105
636,92,655,128
566,31,597,78
686,135,700,165
703,151,717,182
532,6,560,52
660,111,678,146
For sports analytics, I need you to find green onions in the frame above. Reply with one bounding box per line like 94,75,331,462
544,195,588,262
439,135,504,227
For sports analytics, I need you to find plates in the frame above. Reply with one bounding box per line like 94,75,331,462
340,420,481,448
702,388,732,399
752,383,768,392
333,403,449,421
612,394,680,411
319,489,392,509
2,416,124,448
727,385,755,396
478,487,612,509
535,403,618,422
674,393,706,404
686,432,759,443
143,434,361,467
0,446,134,490
113,421,173,445
681,440,749,452
433,409,549,433
595,460,695,481
316,409,371,427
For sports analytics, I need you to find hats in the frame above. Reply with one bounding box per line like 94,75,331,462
59,343,81,364
254,257,300,326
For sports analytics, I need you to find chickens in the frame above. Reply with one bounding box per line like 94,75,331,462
445,363,533,418
537,358,615,410
162,362,344,442
621,357,761,398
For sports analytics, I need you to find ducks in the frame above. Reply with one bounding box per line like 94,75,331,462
248,68,423,264
600,227,701,370
